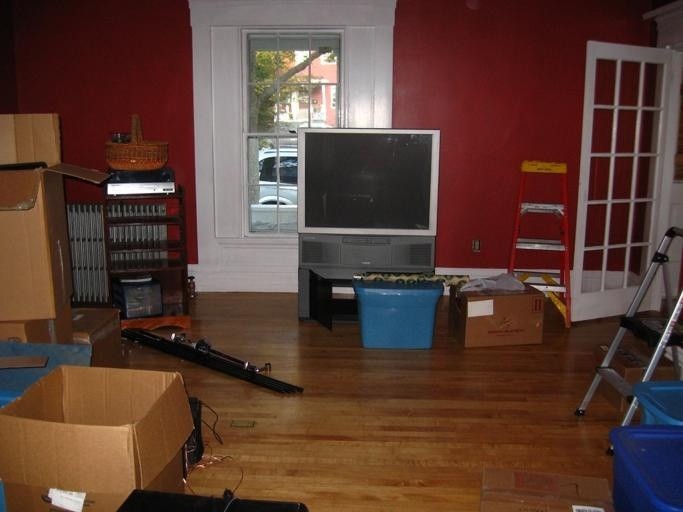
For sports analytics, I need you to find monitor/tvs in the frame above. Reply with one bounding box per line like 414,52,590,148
297,128,441,237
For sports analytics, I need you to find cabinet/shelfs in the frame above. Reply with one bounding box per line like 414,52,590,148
99,186,193,332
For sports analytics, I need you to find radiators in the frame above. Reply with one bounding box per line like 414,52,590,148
65,199,171,311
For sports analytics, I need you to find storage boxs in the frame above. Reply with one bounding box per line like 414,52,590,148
607,381,683,512
0,110,109,320
0,297,78,344
4,362,195,512
351,271,547,350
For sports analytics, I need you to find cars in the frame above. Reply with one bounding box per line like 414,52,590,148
258,147,299,206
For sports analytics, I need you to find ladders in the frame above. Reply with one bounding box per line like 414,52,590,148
506,159,572,328
573,227,683,456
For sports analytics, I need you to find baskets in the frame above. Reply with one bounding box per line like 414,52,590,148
103,113,169,172
